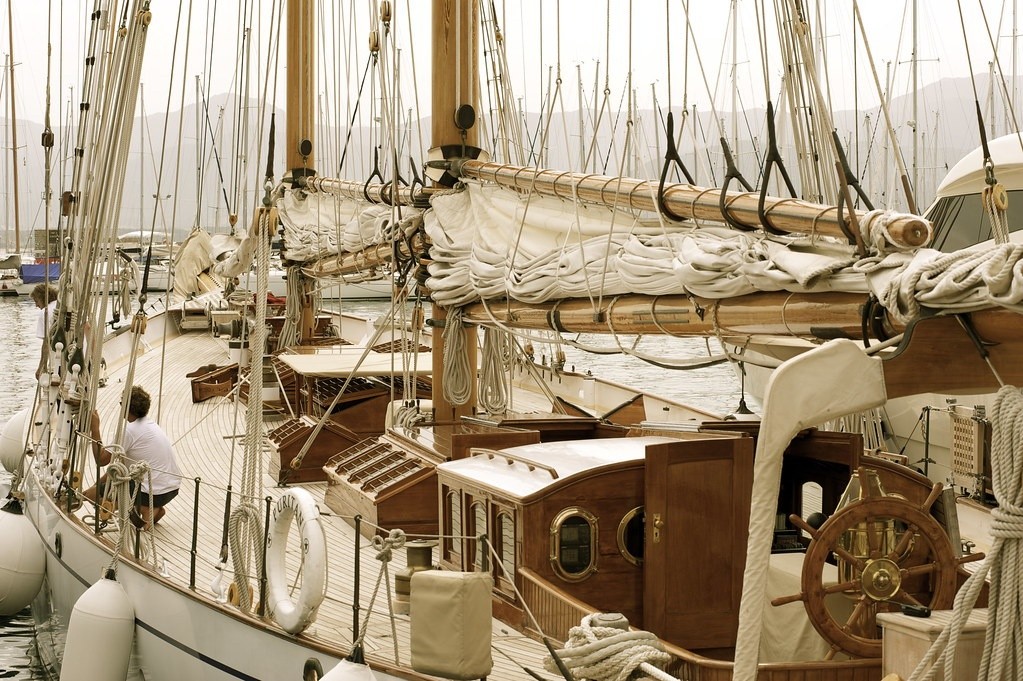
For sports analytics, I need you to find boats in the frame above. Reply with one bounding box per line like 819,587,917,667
0,269,22,295
86,231,185,295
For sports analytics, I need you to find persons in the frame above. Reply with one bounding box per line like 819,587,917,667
89,385,181,530
30,285,87,379
2,282,8,289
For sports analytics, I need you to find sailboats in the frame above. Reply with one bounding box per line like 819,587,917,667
0,1,1023,672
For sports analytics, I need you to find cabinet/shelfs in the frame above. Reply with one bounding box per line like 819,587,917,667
211,311,240,333
876,608,990,681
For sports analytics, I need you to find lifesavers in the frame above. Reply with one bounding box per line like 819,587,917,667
265,487,326,634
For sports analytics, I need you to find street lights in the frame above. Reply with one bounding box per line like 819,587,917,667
152,193,172,231
